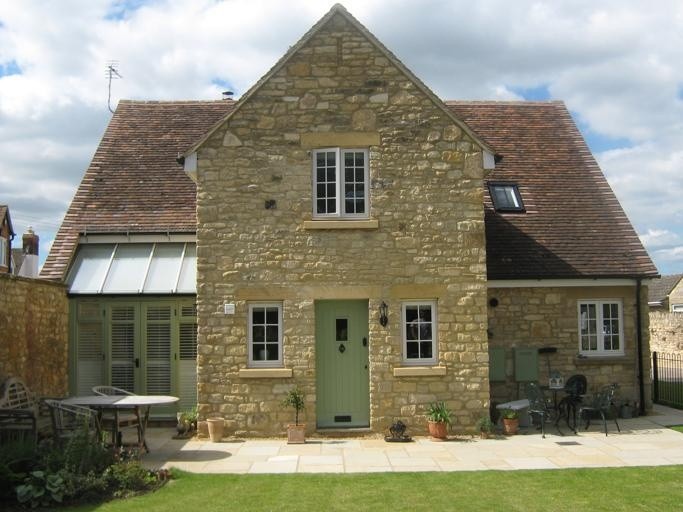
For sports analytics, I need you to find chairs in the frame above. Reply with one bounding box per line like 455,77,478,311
524,372,620,439
43,384,138,451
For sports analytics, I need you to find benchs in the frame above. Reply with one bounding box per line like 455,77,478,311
0,377,65,443
495,399,537,428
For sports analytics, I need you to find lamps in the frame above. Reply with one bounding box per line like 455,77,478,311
377,298,389,328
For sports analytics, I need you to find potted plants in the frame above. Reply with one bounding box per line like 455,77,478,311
425,397,452,442
278,384,310,444
474,406,521,440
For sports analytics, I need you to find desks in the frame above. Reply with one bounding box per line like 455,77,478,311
62,395,179,455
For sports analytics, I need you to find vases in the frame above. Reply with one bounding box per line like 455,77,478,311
206,416,225,442
